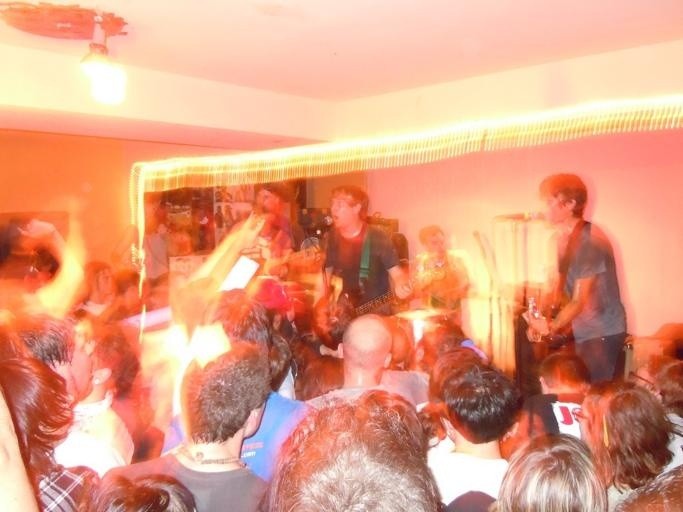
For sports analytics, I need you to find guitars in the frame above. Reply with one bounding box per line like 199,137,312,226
175,244,320,329
528,297,571,348
309,271,446,350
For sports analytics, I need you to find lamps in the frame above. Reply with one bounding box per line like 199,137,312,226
82,14,116,85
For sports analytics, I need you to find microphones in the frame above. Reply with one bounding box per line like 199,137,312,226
310,216,333,230
508,211,544,222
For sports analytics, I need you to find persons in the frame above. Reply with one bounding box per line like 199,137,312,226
0,173,682,512
